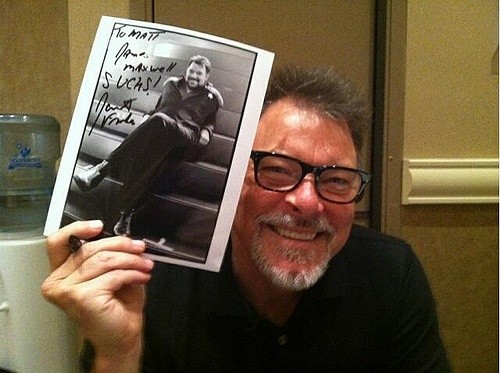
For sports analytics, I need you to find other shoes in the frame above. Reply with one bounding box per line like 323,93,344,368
74,168,102,193
113,208,133,238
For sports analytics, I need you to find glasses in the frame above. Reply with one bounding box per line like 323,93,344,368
250,151,369,206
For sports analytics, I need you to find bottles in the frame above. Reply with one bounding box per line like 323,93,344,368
0,111,61,236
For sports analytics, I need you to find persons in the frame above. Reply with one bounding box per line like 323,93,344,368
41,69,452,373
74,54,223,237
